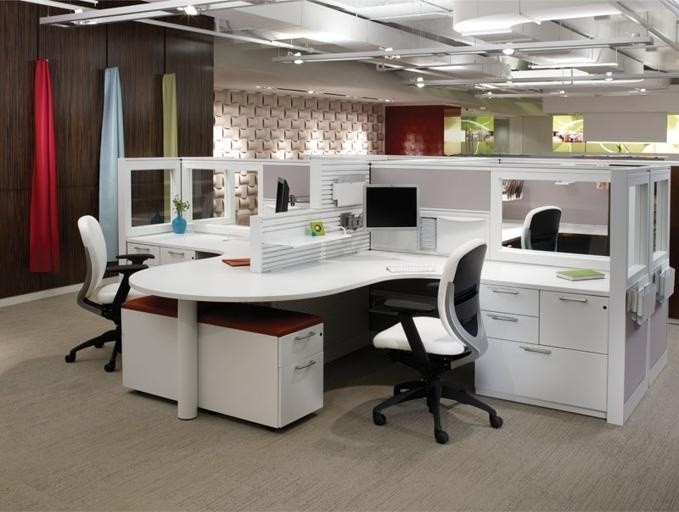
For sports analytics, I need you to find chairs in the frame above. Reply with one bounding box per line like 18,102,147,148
373,239,502,444
65,215,155,372
507,206,562,252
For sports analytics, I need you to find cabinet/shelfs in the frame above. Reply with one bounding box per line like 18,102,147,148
120,295,324,429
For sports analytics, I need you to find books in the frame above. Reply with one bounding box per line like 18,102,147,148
556,269,605,281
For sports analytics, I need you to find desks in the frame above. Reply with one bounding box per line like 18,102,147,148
117,157,676,428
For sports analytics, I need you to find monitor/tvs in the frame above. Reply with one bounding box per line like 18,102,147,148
274,177,291,213
362,182,423,231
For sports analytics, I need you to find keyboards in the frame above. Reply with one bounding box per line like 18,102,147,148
384,262,437,276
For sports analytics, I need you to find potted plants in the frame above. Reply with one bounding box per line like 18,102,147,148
172,198,190,234
